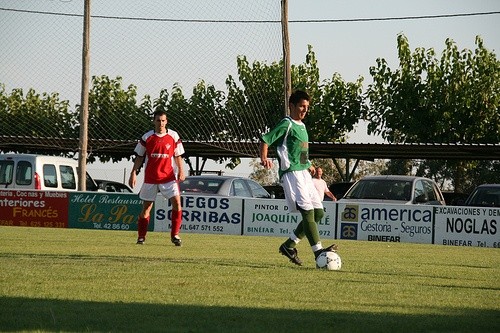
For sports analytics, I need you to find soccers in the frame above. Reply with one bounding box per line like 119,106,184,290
316,251,341,270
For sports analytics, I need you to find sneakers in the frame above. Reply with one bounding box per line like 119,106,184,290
279,244,304,267
314,243,337,260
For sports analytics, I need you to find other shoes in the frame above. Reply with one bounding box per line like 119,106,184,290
171,236,183,245
137,237,146,244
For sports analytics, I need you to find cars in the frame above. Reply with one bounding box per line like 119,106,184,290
338,176,446,205
94,179,133,193
457,184,500,207
179,175,275,200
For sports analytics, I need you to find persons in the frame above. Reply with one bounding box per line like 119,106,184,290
129,108,185,247
260,90,338,266
312,166,337,203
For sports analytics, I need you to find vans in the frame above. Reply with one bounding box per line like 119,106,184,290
0,154,100,192
